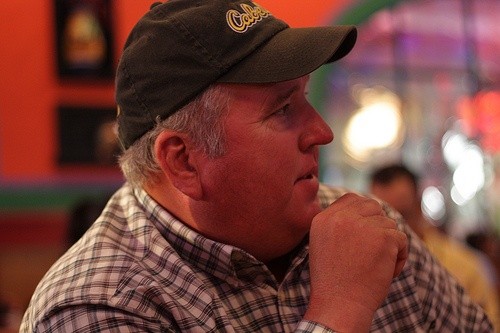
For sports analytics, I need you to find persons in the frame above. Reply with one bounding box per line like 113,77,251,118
369,161,500,333
18,0,495,333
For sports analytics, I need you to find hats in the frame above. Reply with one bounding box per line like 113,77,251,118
116,1,357,150
